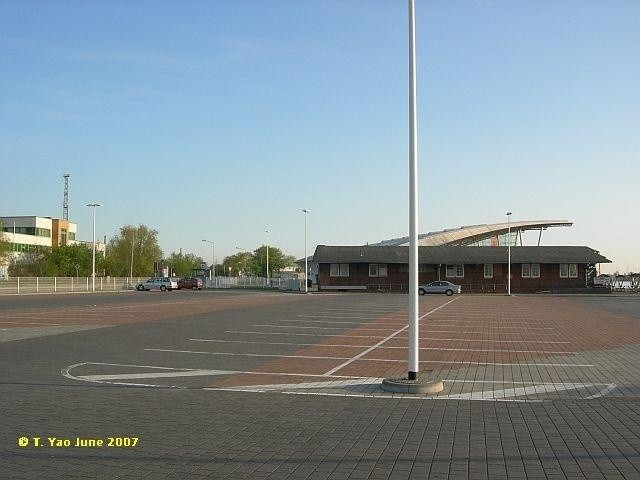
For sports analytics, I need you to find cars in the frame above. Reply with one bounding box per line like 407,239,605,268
136,277,203,291
419,281,462,296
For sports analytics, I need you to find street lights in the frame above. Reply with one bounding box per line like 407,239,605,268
506,212,512,296
265,230,270,286
86,203,103,292
302,209,310,294
201,239,215,277
